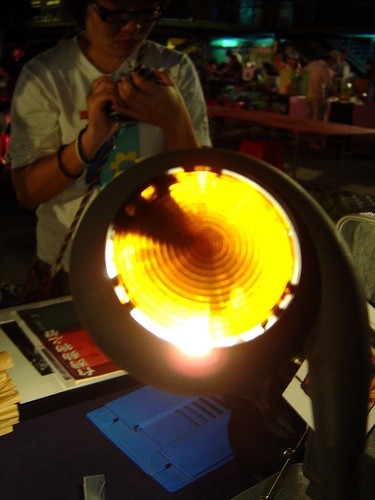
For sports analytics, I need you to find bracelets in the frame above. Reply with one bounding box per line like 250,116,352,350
57,144,84,179
76,127,110,168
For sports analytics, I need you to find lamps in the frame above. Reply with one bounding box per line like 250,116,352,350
71,148,371,500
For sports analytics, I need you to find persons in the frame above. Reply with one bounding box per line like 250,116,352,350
203,37,352,121
9,0,211,299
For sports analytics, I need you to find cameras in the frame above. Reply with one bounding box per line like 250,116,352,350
102,64,163,122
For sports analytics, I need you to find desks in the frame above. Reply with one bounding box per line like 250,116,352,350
207,105,375,179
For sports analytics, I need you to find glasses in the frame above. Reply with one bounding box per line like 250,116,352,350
91,0,163,26
